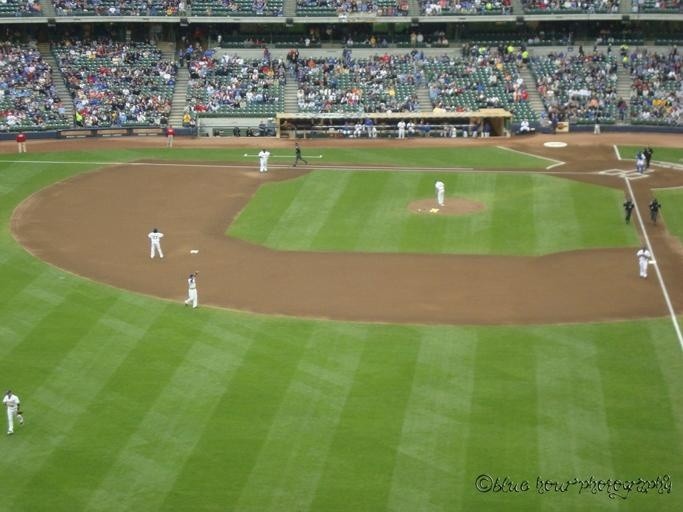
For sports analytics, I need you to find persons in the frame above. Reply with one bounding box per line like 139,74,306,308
622,198,634,224
184,272,198,309
2,389,24,434
0,0,683,150
147,227,165,260
434,180,445,207
258,148,271,174
637,150,646,174
648,198,661,225
636,246,651,280
16,130,27,154
643,146,653,168
292,146,308,167
